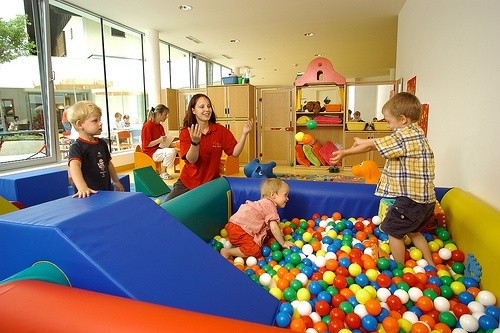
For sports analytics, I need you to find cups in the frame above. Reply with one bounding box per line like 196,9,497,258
245,78,249,84
237,78,243,84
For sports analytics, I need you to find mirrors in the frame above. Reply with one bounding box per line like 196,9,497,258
347,84,396,130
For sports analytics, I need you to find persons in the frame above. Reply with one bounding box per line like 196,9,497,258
164,94,253,202
67,101,124,199
141,104,177,180
348,109,379,130
13,116,20,122
111,111,132,143
220,177,295,260
61,105,71,132
329,92,436,269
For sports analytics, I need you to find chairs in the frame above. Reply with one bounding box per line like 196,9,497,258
58,137,76,160
219,153,239,175
133,145,162,175
111,130,132,152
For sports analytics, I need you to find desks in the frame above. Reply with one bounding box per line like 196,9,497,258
99,124,142,149
165,147,185,177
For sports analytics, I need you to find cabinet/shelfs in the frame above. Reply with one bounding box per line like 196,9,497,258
206,84,254,121
343,132,394,168
215,120,255,166
293,84,346,169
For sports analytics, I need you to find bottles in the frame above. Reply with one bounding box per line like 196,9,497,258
242,78,245,84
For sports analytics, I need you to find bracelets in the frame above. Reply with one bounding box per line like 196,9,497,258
191,140,201,146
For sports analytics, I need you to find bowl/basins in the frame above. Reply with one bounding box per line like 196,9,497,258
345,121,368,131
221,76,238,84
372,122,392,131
324,104,342,112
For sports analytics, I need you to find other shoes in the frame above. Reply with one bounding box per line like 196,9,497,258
159,173,172,180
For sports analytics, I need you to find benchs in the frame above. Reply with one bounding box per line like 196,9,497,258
13,122,30,130
